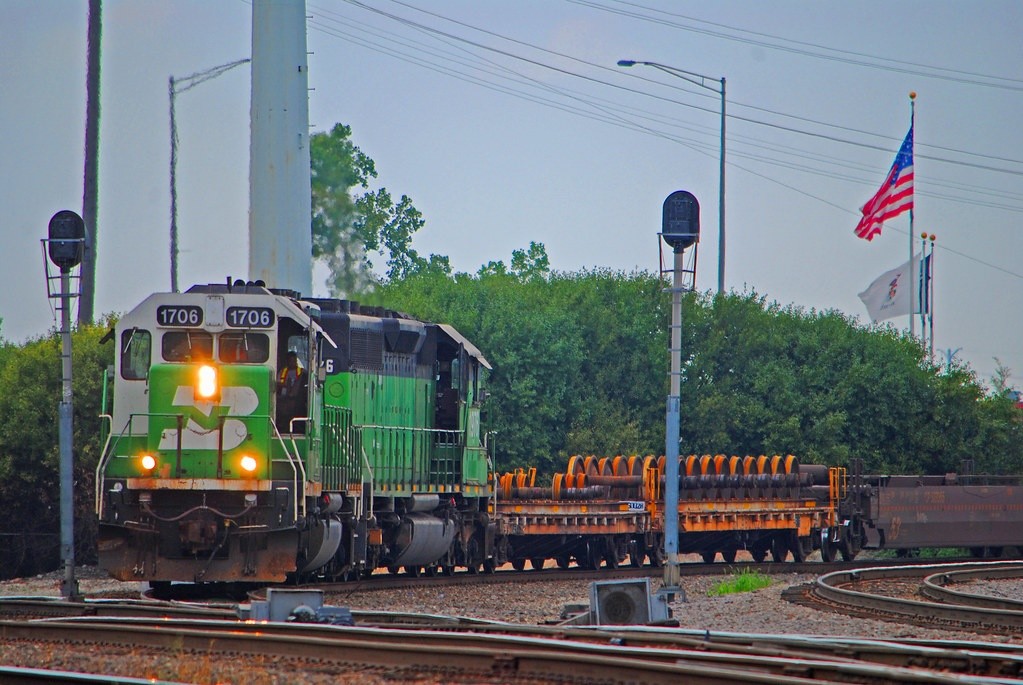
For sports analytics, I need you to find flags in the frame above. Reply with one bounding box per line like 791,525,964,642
858,251,922,324
919,255,930,314
854,122,913,241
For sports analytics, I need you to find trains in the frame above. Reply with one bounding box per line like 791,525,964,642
93,275,1021,596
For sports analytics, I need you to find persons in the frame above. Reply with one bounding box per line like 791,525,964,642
276,351,307,433
